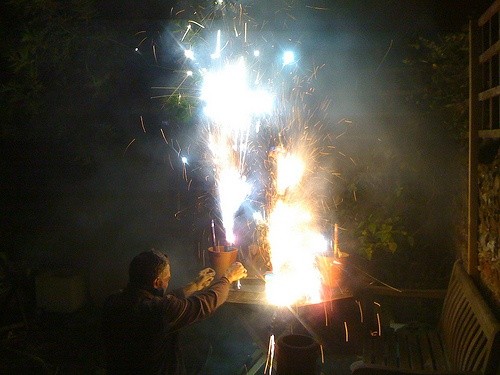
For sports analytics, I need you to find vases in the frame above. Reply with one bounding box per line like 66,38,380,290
209,246,237,277
320,251,349,287
276,332,319,375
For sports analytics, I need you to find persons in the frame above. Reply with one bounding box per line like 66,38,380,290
100,246,248,375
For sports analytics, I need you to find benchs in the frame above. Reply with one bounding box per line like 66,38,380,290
349,260,500,375
